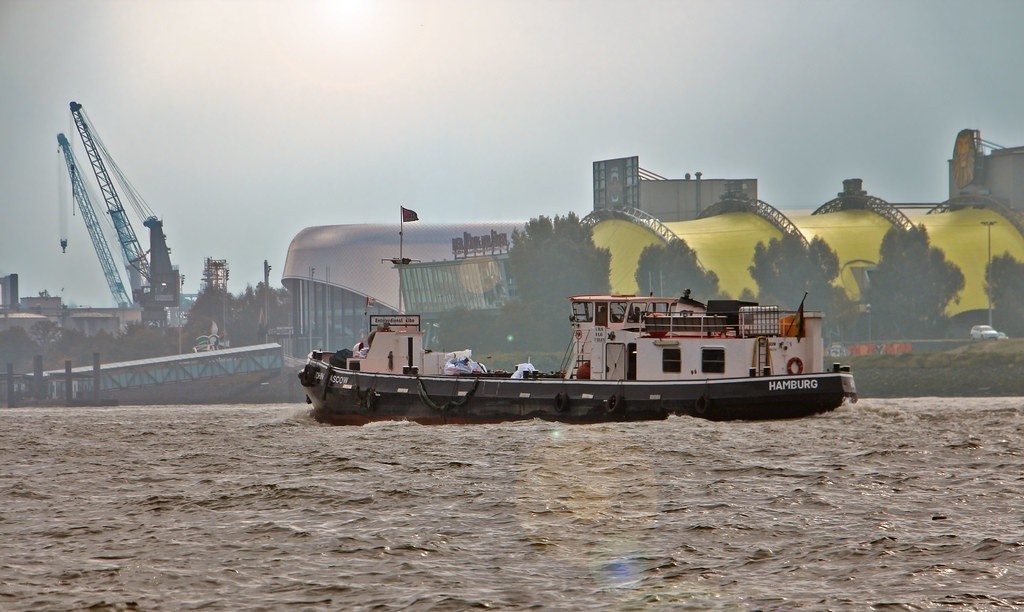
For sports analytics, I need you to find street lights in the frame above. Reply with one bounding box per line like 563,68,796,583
981,221,997,326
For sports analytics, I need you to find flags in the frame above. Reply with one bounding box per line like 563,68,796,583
403,208,419,222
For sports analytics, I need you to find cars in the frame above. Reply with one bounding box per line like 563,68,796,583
970,325,998,340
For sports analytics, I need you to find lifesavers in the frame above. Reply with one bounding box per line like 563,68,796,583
552,390,571,413
785,357,805,376
605,392,623,415
693,392,710,417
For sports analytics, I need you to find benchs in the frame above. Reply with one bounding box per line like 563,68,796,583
644,315,728,338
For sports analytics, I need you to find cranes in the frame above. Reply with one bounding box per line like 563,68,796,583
56,133,132,308
69,102,181,321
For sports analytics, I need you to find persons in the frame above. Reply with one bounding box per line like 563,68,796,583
633,307,640,321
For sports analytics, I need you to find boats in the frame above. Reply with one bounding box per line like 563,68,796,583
297,204,859,426
